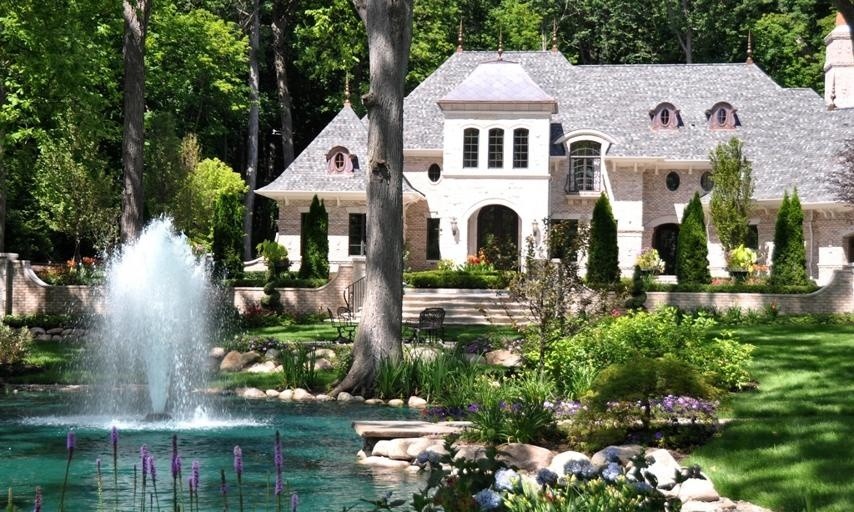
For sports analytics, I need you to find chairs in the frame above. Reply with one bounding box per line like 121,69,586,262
326,306,445,346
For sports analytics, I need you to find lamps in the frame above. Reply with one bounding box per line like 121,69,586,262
531,218,538,236
450,215,457,236
